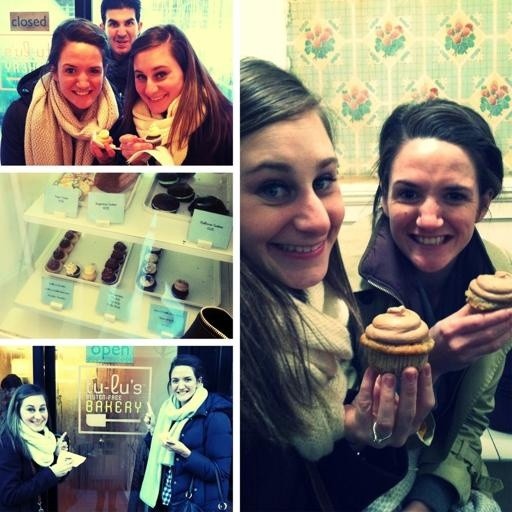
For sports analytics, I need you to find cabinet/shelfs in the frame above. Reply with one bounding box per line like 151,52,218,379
0,174,233,340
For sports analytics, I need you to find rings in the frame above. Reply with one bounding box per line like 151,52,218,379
372,422,393,445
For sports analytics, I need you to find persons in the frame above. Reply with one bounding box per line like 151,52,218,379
0,384,74,512
1,18,128,166
241,59,436,511
135,353,233,512
118,23,233,165
0,374,22,399
353,99,512,511
100,1,143,95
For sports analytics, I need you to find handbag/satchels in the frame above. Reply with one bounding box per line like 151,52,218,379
179,462,232,512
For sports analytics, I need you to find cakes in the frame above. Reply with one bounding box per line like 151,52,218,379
149,245,159,253
145,128,161,146
464,270,512,313
145,253,158,263
94,130,114,145
65,262,80,278
171,278,190,298
82,262,97,281
359,305,434,376
45,229,82,273
138,275,156,291
102,242,127,285
142,262,157,275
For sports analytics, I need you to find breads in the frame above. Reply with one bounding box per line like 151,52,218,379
94,173,140,192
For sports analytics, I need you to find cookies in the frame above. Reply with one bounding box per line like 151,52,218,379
178,173,194,179
152,193,180,213
188,196,229,218
168,182,195,201
158,174,180,188
